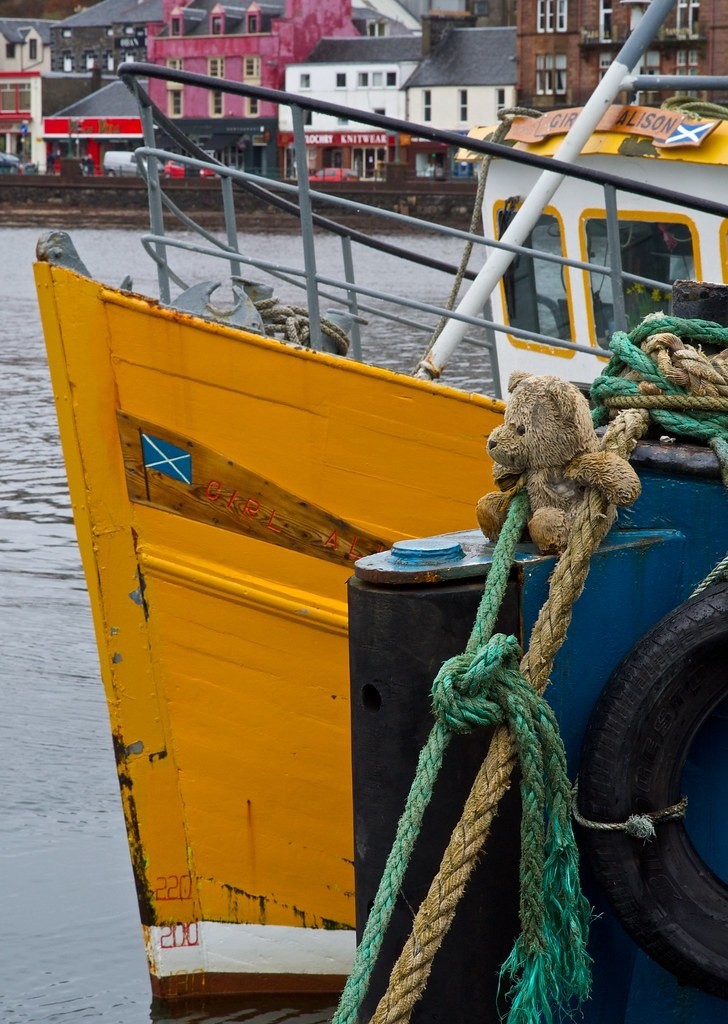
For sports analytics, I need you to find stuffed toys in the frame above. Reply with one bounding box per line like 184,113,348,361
474,370,642,553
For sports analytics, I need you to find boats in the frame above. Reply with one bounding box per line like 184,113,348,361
32,102,726,1021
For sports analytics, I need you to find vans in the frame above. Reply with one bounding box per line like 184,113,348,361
101,149,166,178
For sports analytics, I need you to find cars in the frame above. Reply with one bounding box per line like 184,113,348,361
304,166,358,183
164,158,216,178
0,151,23,174
